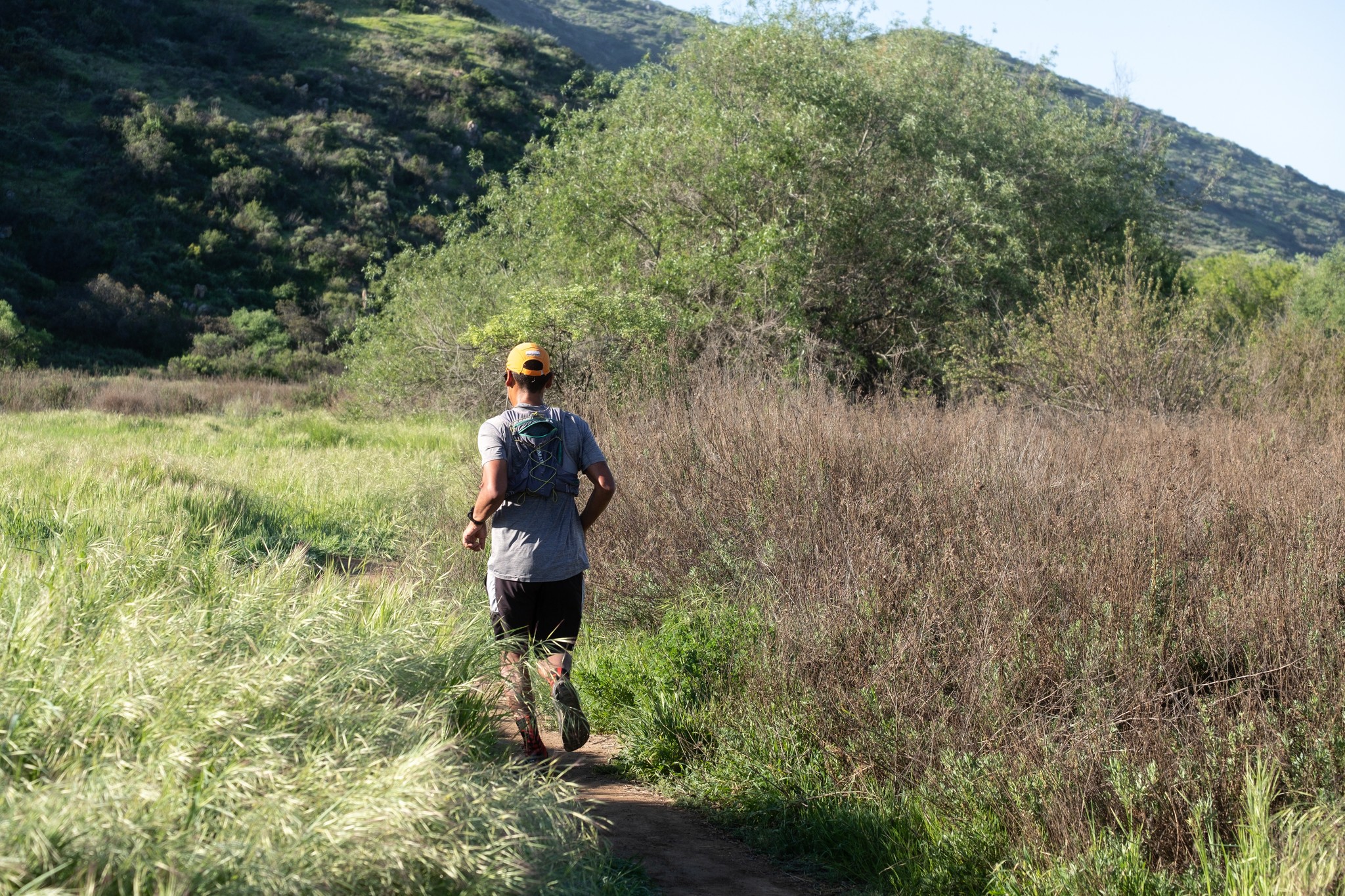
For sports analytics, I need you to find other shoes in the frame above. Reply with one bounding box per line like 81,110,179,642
553,681,590,752
524,752,550,775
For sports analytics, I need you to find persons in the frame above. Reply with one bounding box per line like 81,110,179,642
463,342,616,773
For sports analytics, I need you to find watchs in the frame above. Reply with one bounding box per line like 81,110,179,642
467,506,485,526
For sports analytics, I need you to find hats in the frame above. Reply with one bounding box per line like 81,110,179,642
505,342,550,377
